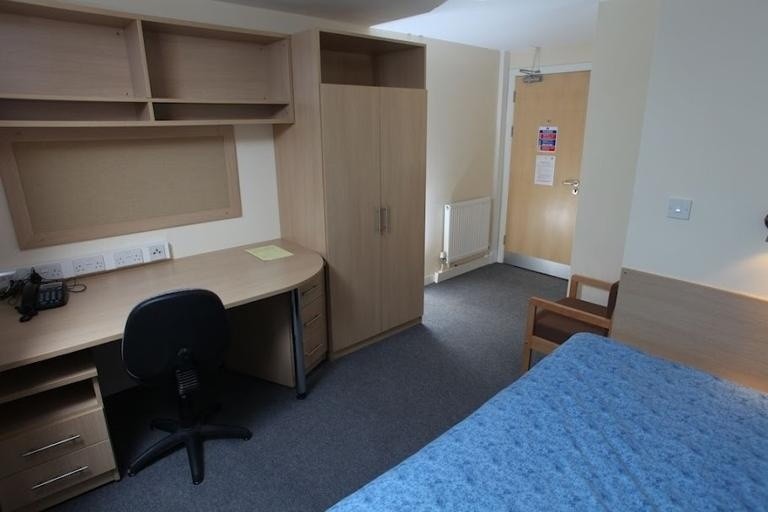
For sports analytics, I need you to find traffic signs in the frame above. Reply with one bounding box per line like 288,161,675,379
50,244,168,282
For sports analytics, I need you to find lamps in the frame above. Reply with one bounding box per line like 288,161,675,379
519,275,619,373
118,289,256,485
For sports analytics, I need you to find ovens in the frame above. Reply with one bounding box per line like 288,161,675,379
325,330,768,512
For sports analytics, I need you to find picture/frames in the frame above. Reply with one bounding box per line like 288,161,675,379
0,240,335,506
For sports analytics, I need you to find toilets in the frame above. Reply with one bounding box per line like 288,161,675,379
20,278,67,314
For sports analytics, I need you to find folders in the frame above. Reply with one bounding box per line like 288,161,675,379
441,197,494,265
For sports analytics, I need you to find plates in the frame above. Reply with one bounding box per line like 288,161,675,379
273,28,428,360
0,2,297,134
